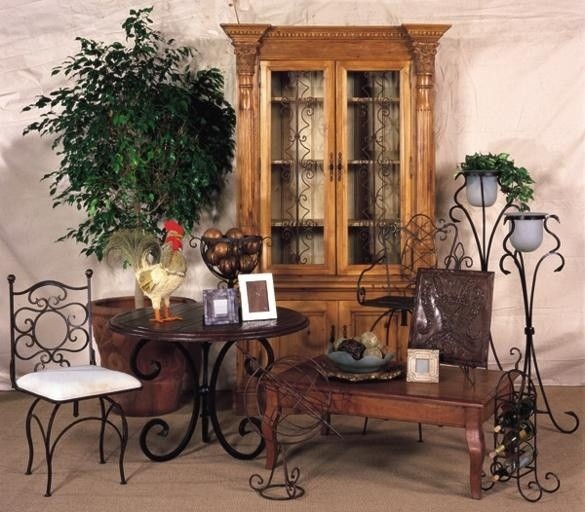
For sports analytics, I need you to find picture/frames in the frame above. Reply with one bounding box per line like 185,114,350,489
203,273,277,326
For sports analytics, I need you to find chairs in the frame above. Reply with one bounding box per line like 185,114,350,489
7,268,145,498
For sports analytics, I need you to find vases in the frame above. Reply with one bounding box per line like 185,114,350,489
506,212,549,252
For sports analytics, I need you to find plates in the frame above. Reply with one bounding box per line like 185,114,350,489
325,345,396,373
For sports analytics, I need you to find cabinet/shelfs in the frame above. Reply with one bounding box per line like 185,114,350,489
220,24,453,415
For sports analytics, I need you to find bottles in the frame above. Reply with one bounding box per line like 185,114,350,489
489,398,538,483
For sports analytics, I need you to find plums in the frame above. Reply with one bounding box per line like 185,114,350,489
204,228,259,274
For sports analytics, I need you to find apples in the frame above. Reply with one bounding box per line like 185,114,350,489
333,331,388,358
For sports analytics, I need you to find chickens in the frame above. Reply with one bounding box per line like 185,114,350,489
104,219,185,322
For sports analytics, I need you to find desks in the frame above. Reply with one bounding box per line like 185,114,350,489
111,304,308,461
260,353,520,500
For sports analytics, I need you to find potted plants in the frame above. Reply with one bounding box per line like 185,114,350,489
20,7,237,416
462,151,535,211
